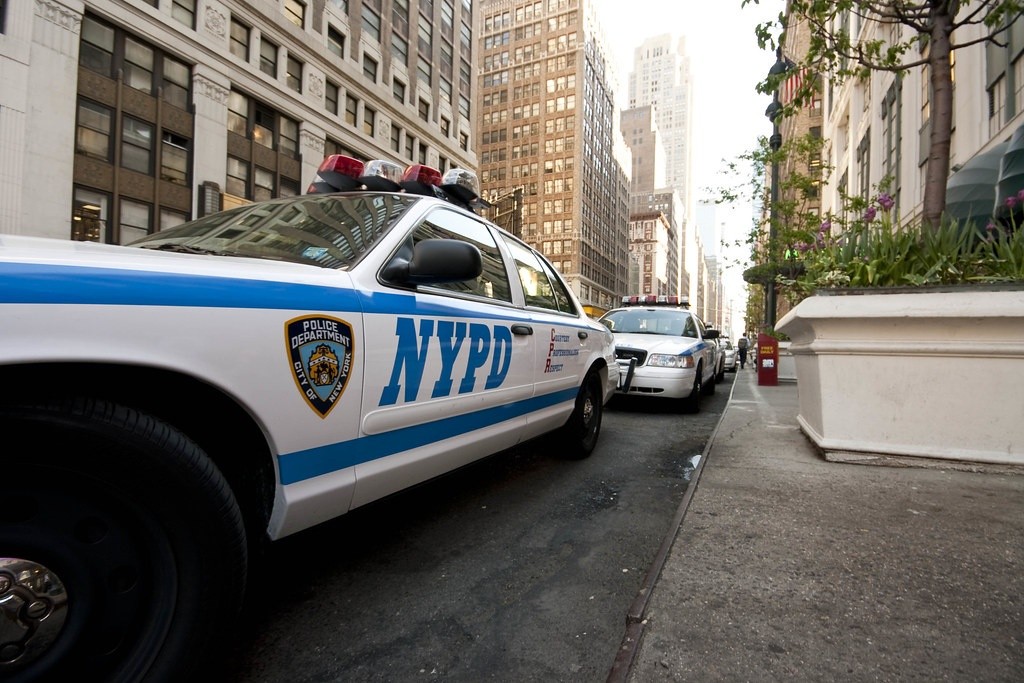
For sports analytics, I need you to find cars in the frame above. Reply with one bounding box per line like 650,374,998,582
0,151,610,683
719,334,738,374
595,293,717,408
708,330,726,383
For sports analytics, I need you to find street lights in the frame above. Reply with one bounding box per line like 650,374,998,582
765,47,789,334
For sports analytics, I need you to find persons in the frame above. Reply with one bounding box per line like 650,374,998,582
738,332,750,369
